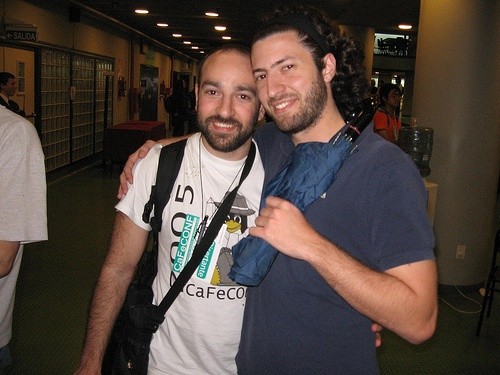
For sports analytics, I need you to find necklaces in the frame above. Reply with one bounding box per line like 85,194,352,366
199,130,248,247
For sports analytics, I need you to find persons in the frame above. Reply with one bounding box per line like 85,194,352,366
118,6,438,375
0,71,49,375
73,41,383,375
170,78,404,145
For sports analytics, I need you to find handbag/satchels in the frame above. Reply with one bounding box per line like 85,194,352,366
100,240,166,375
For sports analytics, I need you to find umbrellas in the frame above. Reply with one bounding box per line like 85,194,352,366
226,106,375,287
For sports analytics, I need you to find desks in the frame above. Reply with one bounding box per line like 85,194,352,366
103,120,166,178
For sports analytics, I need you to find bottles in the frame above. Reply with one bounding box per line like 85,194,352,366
398,127,433,178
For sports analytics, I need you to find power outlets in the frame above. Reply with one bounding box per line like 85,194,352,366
456,246,464,260
